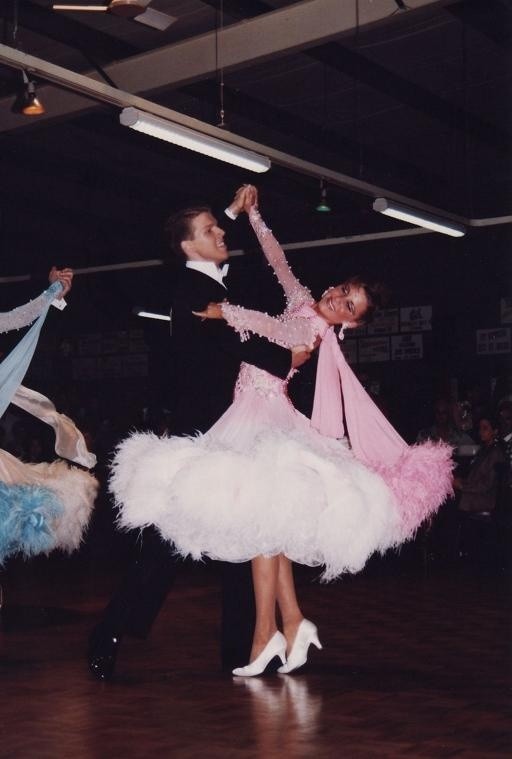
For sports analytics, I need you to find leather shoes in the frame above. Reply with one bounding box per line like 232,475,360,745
86,622,121,683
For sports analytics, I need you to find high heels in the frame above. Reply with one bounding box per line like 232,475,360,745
231,630,287,677
276,618,323,674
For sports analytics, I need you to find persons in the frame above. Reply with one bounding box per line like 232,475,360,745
109,182,450,680
0,266,98,572
84,182,310,680
399,370,512,570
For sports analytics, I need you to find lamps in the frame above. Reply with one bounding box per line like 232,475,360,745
118,105,273,177
371,197,470,242
